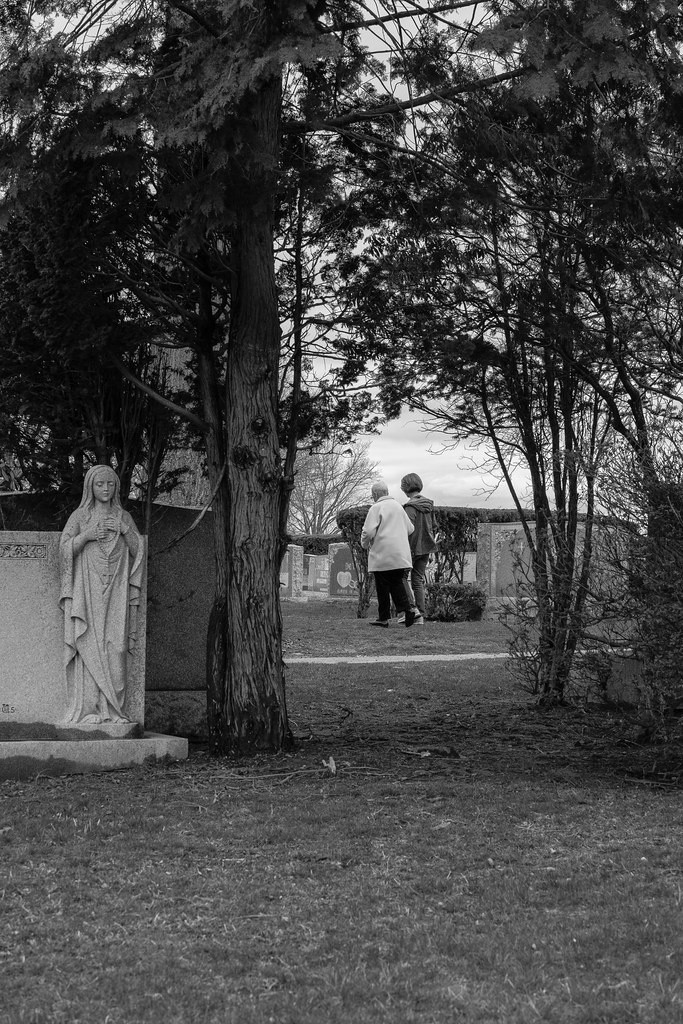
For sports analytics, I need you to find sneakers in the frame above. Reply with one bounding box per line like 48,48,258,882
398,607,421,623
413,616,423,624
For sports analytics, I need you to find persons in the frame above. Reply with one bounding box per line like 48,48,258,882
360,473,439,627
59,464,138,725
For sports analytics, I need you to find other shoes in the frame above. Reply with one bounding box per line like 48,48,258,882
405,611,415,627
369,620,388,628
